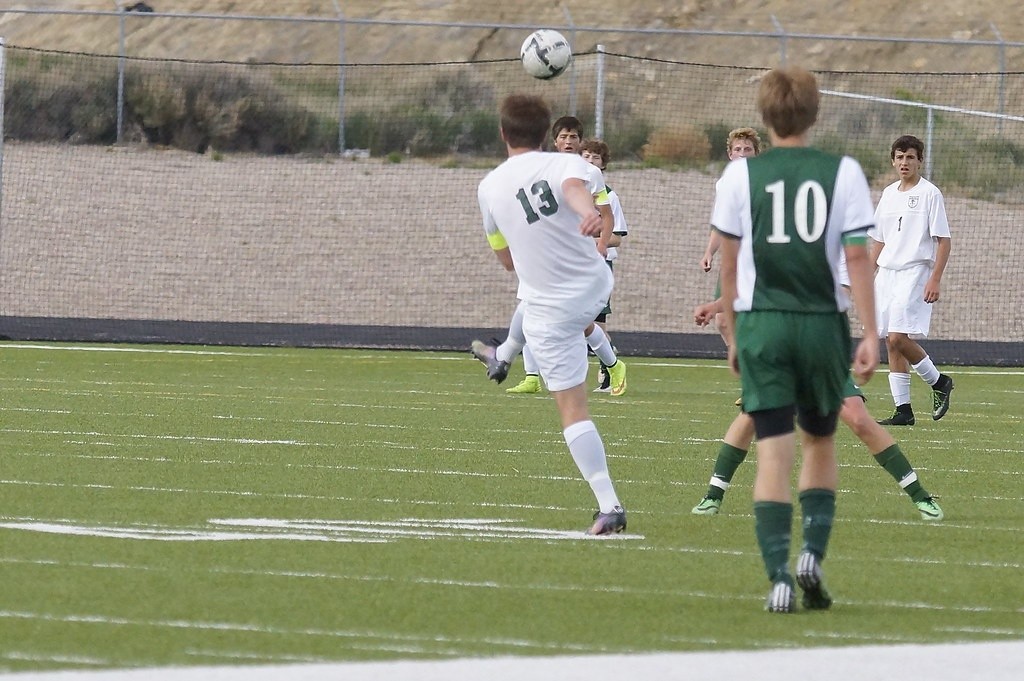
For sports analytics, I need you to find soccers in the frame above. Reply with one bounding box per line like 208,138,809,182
518,27,573,80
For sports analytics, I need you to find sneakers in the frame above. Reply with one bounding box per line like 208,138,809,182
795,554,832,611
764,583,794,613
470,338,511,385
929,376,953,421
876,410,915,426
691,496,722,514
606,360,627,396
734,397,742,406
915,496,944,521
586,505,627,535
505,375,542,393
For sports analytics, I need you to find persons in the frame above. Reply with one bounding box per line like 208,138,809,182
506,116,629,396
867,135,954,425
700,127,762,406
711,68,879,614
578,140,628,392
692,242,944,520
470,94,628,535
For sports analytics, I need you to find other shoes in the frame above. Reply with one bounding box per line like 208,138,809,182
599,361,610,389
